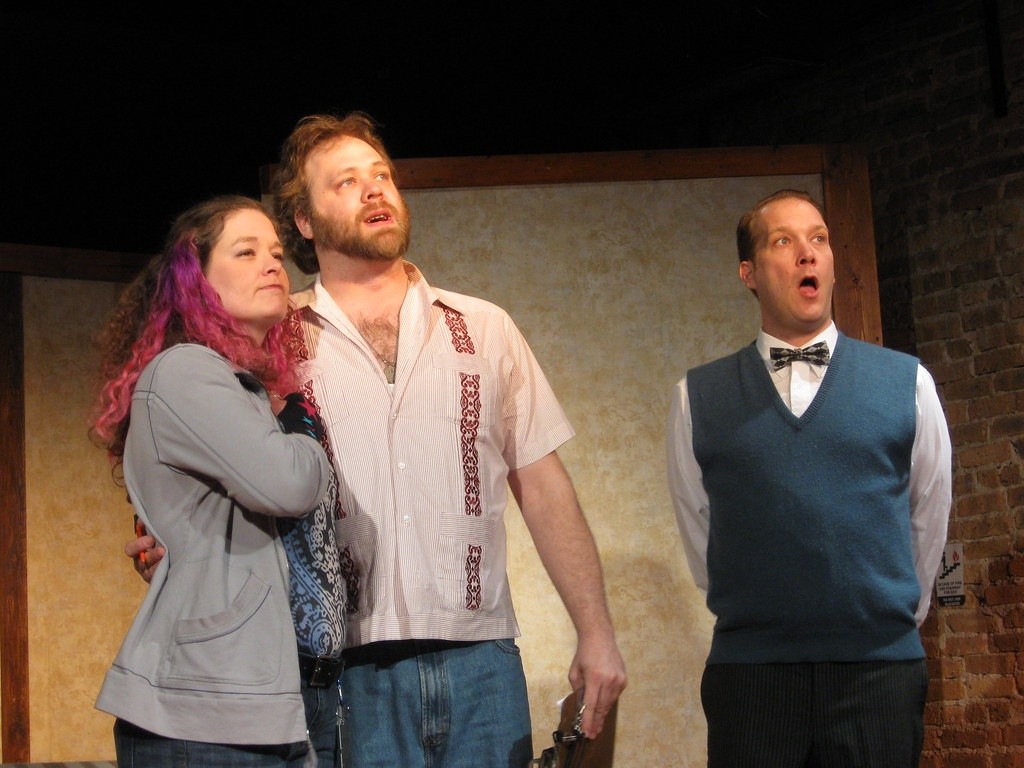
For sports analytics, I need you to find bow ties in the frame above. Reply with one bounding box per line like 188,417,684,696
770,340,831,371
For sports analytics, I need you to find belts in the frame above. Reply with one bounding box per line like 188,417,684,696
297,651,345,688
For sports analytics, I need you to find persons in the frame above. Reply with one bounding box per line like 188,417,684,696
666,189,953,768
89,197,379,768
126,116,627,768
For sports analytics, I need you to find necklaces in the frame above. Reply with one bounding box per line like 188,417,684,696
353,326,397,384
